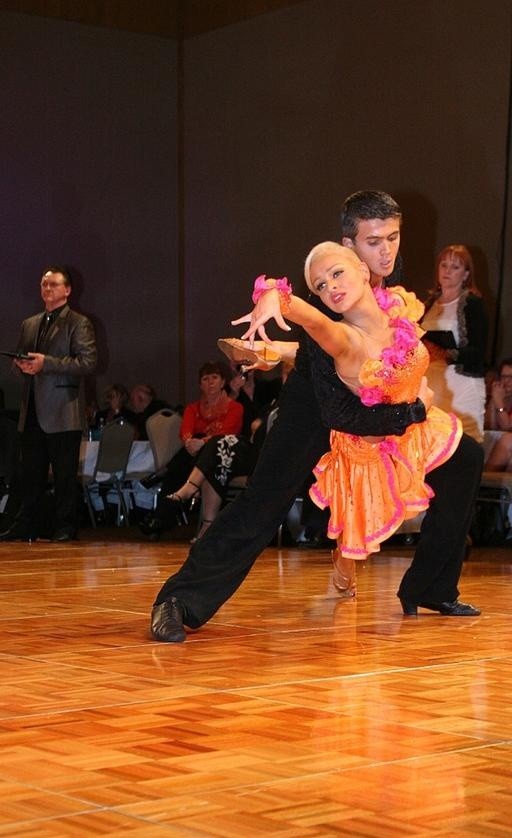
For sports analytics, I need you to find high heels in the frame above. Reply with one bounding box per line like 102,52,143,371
331,549,357,598
165,480,201,511
188,520,214,544
218,338,280,374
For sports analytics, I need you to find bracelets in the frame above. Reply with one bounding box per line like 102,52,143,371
497,408,506,411
252,274,292,313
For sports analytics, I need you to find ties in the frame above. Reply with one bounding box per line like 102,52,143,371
38,312,53,348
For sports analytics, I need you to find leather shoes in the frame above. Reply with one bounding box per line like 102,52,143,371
0,531,33,543
51,532,76,541
139,472,160,490
151,596,186,641
400,597,481,616
138,519,161,535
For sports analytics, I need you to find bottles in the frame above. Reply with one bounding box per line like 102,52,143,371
87,408,121,441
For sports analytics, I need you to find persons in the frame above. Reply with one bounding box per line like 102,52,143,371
147,186,485,644
97,383,153,441
227,237,463,600
8,263,101,544
417,243,488,377
166,363,282,547
483,360,512,472
